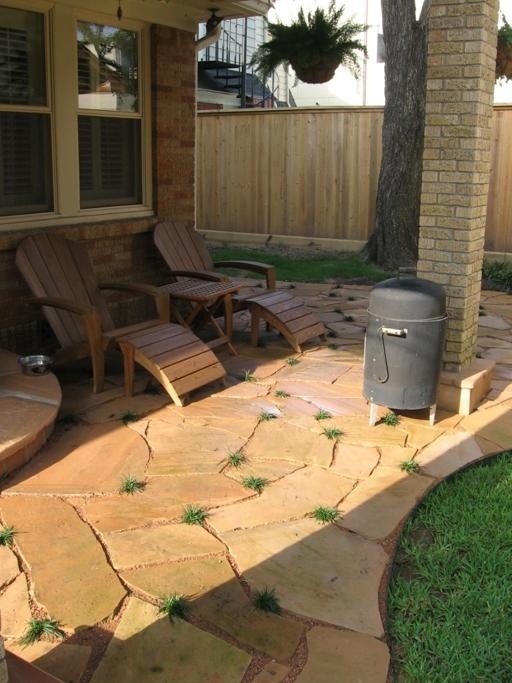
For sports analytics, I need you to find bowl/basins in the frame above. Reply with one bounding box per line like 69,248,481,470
18,355,54,377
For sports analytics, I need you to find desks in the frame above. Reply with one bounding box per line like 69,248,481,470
160,278,244,356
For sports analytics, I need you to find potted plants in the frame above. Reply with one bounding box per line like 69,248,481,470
495,9,512,84
245,0,369,84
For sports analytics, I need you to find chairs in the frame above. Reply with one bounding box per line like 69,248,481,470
15,232,227,408
153,218,329,354
